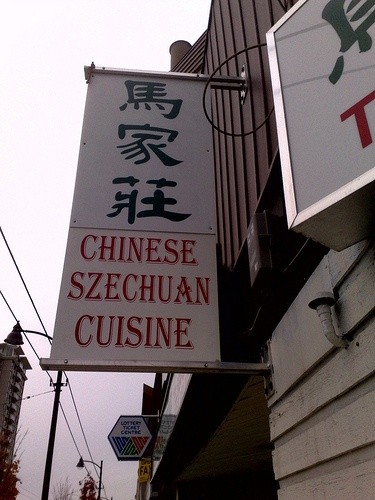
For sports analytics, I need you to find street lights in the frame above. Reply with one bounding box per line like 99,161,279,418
75,455,103,500
5,320,64,500
